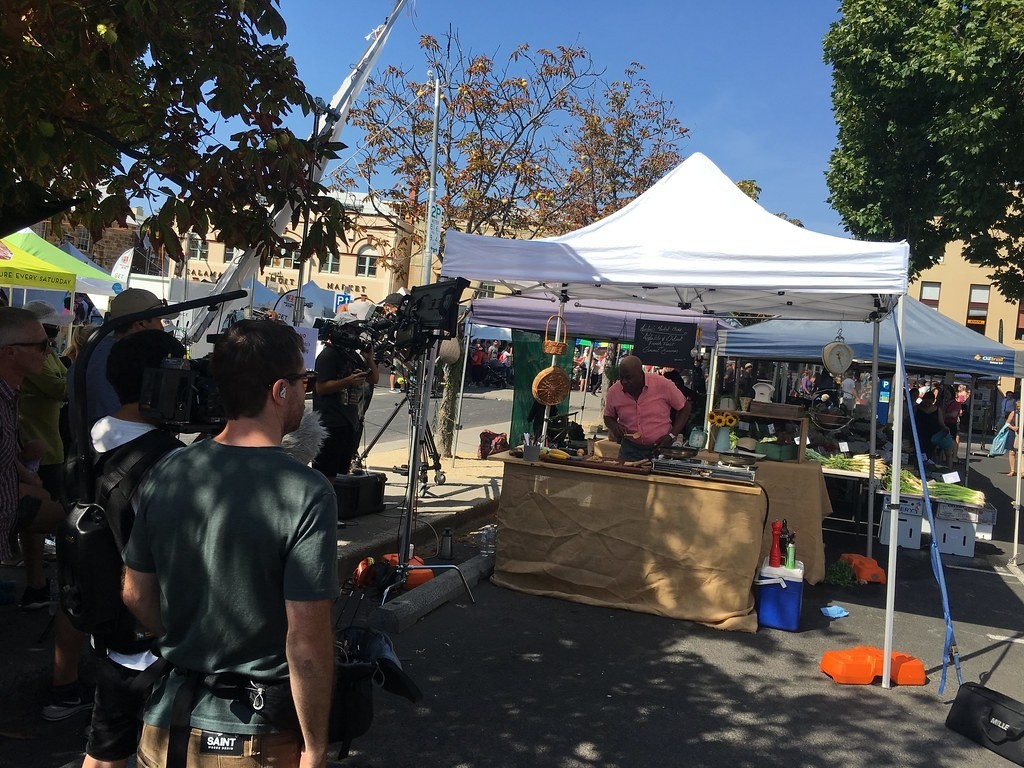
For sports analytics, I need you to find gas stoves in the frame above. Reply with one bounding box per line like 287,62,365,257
651,458,756,486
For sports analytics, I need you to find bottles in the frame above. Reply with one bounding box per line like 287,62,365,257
438,526,452,560
480,526,489,557
769,519,797,569
487,523,496,554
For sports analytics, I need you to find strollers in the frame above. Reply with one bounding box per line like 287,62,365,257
571,366,587,390
483,361,507,389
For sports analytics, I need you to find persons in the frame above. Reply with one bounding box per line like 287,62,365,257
999,391,1024,478
123,324,339,768
390,357,412,394
786,366,872,413
475,338,513,384
903,379,969,468
312,312,380,527
571,346,628,397
351,292,404,475
0,290,204,768
604,355,692,461
645,362,751,435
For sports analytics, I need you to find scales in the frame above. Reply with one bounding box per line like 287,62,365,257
752,379,775,404
809,328,857,432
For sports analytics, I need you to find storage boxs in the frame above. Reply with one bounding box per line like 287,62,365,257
757,556,804,632
876,480,998,557
326,471,388,520
755,438,798,462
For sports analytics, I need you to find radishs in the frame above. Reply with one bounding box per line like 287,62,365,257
852,454,886,475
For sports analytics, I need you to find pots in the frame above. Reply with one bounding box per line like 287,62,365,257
719,452,756,465
658,446,699,458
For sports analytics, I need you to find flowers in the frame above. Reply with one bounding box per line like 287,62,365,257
810,393,841,414
707,409,740,427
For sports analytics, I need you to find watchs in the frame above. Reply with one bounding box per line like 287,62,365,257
668,432,677,442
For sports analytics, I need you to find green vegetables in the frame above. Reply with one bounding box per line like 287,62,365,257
880,464,924,495
805,447,853,470
825,560,856,586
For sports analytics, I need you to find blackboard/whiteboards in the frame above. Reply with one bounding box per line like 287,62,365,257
633,319,697,370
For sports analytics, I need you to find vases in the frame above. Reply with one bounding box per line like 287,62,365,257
813,412,850,423
713,428,729,453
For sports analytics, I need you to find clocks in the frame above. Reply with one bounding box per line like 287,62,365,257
822,329,853,378
689,426,708,450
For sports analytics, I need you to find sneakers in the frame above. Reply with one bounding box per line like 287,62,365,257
0,546,97,722
389,388,399,394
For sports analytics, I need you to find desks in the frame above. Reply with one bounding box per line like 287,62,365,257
594,438,821,579
487,445,762,620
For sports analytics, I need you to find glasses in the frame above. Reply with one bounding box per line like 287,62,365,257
265,371,319,392
146,298,168,312
2,338,49,355
43,326,58,337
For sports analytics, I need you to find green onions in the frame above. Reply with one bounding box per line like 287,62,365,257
927,481,986,507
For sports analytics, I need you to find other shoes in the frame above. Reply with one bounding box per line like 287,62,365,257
1021,472,1024,478
1008,471,1015,476
590,392,598,397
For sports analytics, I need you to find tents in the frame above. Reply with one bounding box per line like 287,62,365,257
0,227,127,348
703,294,1024,566
451,291,740,468
398,152,909,690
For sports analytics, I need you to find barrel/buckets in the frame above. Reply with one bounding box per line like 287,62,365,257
931,426,953,450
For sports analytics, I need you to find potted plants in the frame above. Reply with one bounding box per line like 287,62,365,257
714,394,739,411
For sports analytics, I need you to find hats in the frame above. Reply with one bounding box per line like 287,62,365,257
745,363,753,369
110,288,179,320
385,293,407,305
21,300,75,326
332,312,363,327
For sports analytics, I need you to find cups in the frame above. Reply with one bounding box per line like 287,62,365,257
408,544,414,560
523,444,540,462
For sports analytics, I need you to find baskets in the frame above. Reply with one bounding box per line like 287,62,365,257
533,366,571,406
543,315,567,354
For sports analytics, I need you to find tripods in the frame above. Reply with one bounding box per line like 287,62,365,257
355,369,446,498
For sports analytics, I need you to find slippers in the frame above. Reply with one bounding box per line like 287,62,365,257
953,458,964,465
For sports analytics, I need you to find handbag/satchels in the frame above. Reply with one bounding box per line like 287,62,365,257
990,423,1010,456
1005,411,1011,418
57,455,95,516
249,624,421,742
1013,434,1024,452
931,428,953,449
948,682,1024,768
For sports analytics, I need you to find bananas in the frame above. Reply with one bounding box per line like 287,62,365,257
545,449,571,460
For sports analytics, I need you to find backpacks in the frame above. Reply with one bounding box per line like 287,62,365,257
499,350,508,363
55,439,188,652
